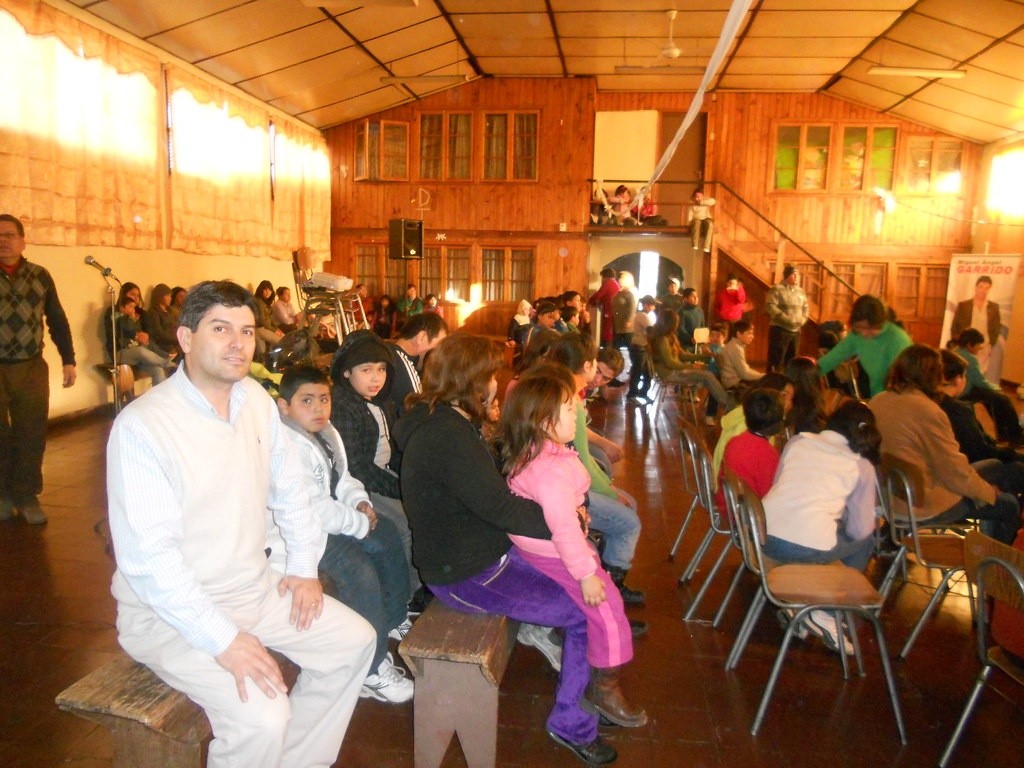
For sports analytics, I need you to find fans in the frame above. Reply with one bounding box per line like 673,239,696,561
632,10,708,71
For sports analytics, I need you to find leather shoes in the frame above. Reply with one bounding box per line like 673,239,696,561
597,715,615,728
546,725,618,766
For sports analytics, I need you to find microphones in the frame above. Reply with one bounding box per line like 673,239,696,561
84,255,116,280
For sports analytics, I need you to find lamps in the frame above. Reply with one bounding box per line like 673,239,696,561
380,41,468,84
867,37,967,78
615,36,708,74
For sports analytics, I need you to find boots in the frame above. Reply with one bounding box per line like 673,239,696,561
602,561,645,602
629,621,648,636
580,667,648,728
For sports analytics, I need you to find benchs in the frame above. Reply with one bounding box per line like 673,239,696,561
503,342,521,368
96,362,150,408
397,595,518,768
54,571,333,768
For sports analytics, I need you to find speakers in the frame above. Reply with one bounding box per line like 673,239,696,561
388,218,424,259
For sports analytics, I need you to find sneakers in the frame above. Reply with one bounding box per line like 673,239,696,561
386,619,413,641
776,608,809,640
802,609,855,656
516,623,562,672
359,652,415,705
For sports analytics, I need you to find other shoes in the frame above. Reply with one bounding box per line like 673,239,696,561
703,248,709,252
167,353,177,360
705,415,715,426
638,390,653,403
626,395,641,406
405,598,427,615
608,378,625,387
0,498,14,520
17,500,46,525
687,396,700,402
974,600,994,625
591,394,608,405
693,246,698,250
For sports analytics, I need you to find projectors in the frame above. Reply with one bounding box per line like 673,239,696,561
311,271,354,292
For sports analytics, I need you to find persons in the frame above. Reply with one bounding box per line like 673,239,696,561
486,374,648,726
329,329,434,615
353,284,443,339
547,332,648,637
268,364,414,704
387,312,450,404
591,185,666,226
713,295,1024,655
0,214,76,524
106,280,377,768
251,280,303,354
484,397,500,424
688,189,716,252
105,282,188,387
951,276,1006,373
507,361,576,671
578,346,624,479
392,332,619,766
588,267,746,425
765,265,809,373
506,291,591,396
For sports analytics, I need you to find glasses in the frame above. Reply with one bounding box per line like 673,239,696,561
0,232,20,239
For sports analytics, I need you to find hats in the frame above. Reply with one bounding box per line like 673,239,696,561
667,277,680,288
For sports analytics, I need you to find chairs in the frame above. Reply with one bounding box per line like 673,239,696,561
293,247,370,347
645,328,1024,767
